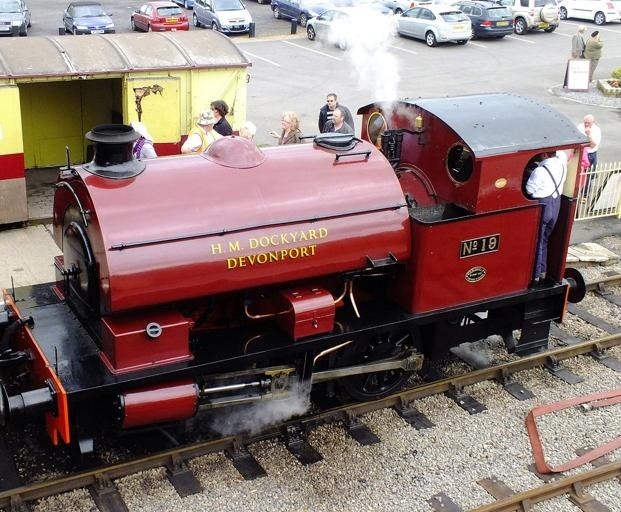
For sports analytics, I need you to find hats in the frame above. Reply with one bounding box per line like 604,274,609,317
130,123,151,141
197,111,216,125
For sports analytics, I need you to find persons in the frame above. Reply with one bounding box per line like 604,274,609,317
526,148,569,286
322,107,355,135
579,146,591,201
237,120,266,149
572,25,587,60
269,112,307,147
584,30,605,84
206,98,233,137
318,93,355,135
577,114,603,180
128,120,158,159
180,111,221,154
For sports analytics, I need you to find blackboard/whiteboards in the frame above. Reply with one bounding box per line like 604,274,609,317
567,59,590,93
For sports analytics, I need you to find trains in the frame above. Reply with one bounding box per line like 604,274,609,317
0,91,592,476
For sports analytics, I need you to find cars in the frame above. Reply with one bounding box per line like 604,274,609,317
558,1,621,26
63,1,117,35
170,0,194,9
130,1,190,32
254,0,417,52
394,4,474,47
0,0,32,37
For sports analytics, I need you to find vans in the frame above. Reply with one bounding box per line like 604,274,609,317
193,0,253,34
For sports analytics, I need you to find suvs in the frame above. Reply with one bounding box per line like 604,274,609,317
450,0,515,41
499,1,561,35
